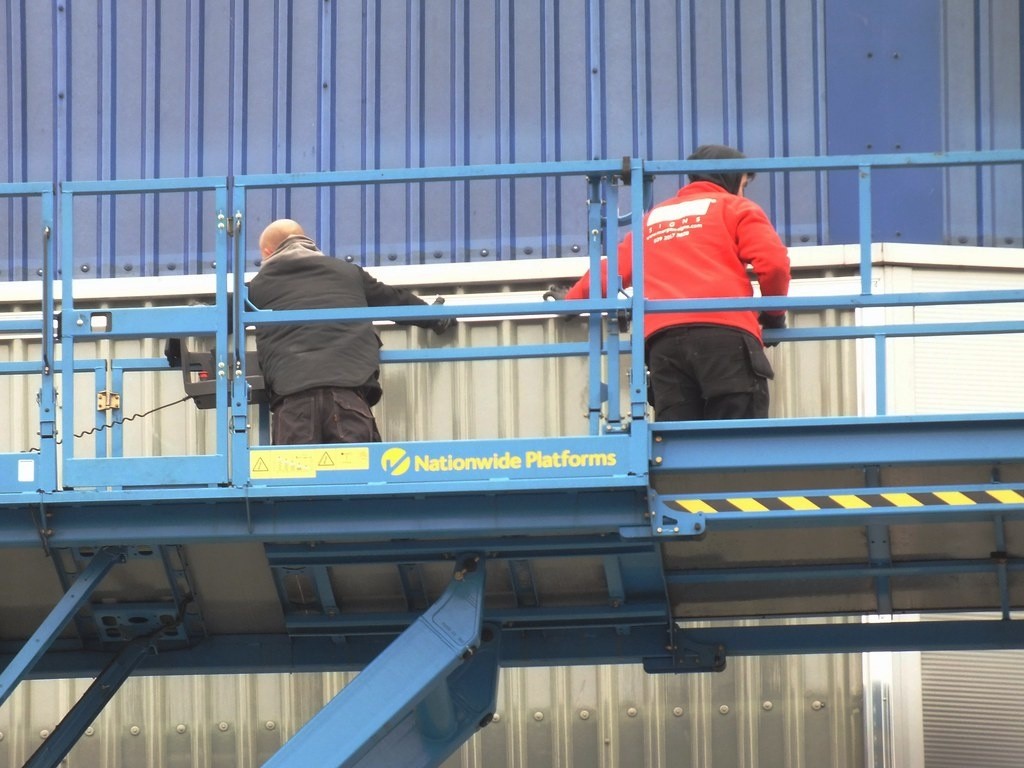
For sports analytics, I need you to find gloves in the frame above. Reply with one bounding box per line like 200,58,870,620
544,284,577,322
758,310,786,348
431,297,458,334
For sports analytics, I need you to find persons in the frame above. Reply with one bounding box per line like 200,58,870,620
165,219,458,445
544,145,790,422
